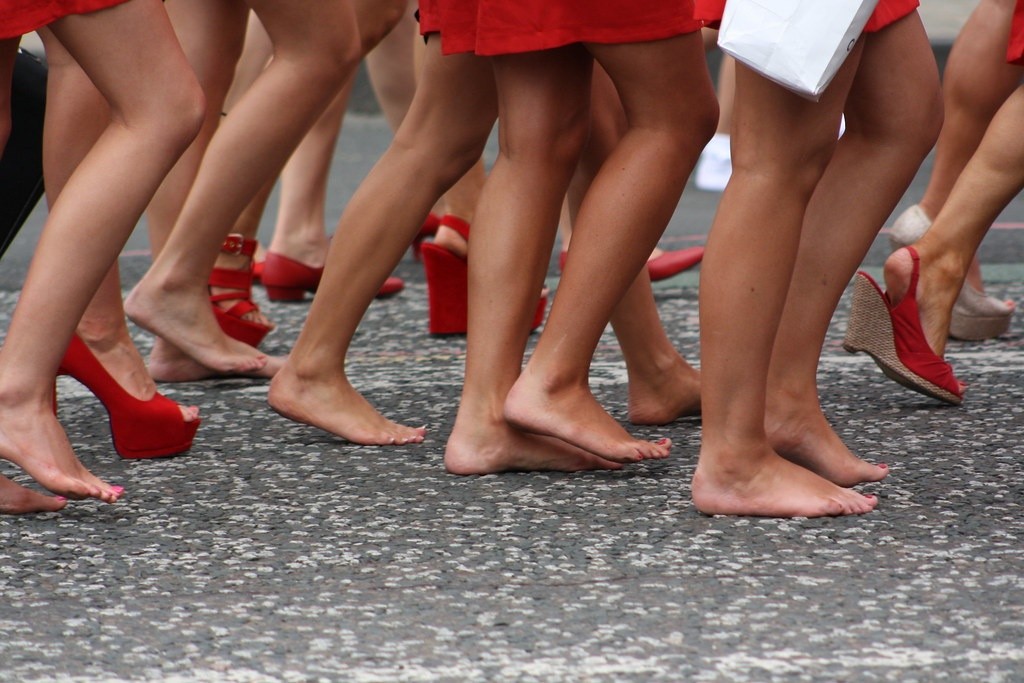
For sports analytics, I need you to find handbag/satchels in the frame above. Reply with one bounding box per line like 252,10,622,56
717,0,881,104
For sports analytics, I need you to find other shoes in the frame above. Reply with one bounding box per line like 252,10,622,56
695,133,733,193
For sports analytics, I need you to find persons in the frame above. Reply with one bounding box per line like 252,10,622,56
0,0,1024,516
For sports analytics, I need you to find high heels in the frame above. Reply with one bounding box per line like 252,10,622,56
261,250,405,303
889,204,1012,340
52,329,200,461
207,233,271,349
559,247,705,280
423,213,549,338
843,245,965,404
